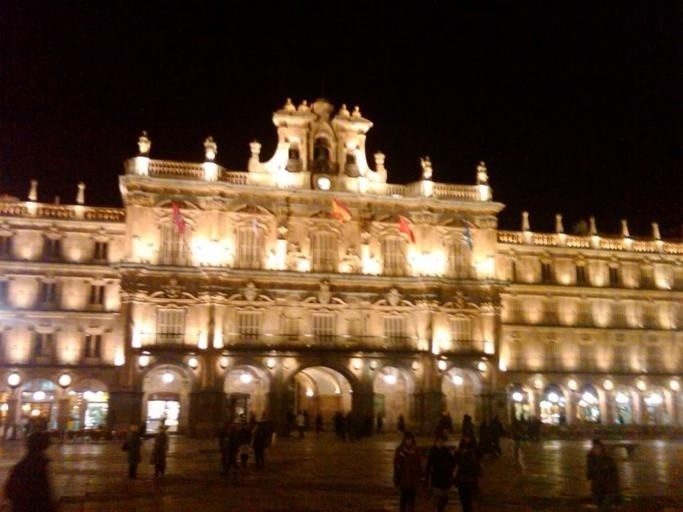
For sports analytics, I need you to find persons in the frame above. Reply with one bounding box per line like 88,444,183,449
5,432,52,512
586,437,621,512
438,411,542,455
392,430,480,511
123,421,168,482
217,413,272,479
283,410,372,442
398,414,406,433
376,411,384,434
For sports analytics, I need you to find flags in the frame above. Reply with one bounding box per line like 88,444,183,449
399,216,416,245
252,205,258,238
463,219,473,250
170,200,186,234
330,195,354,222
